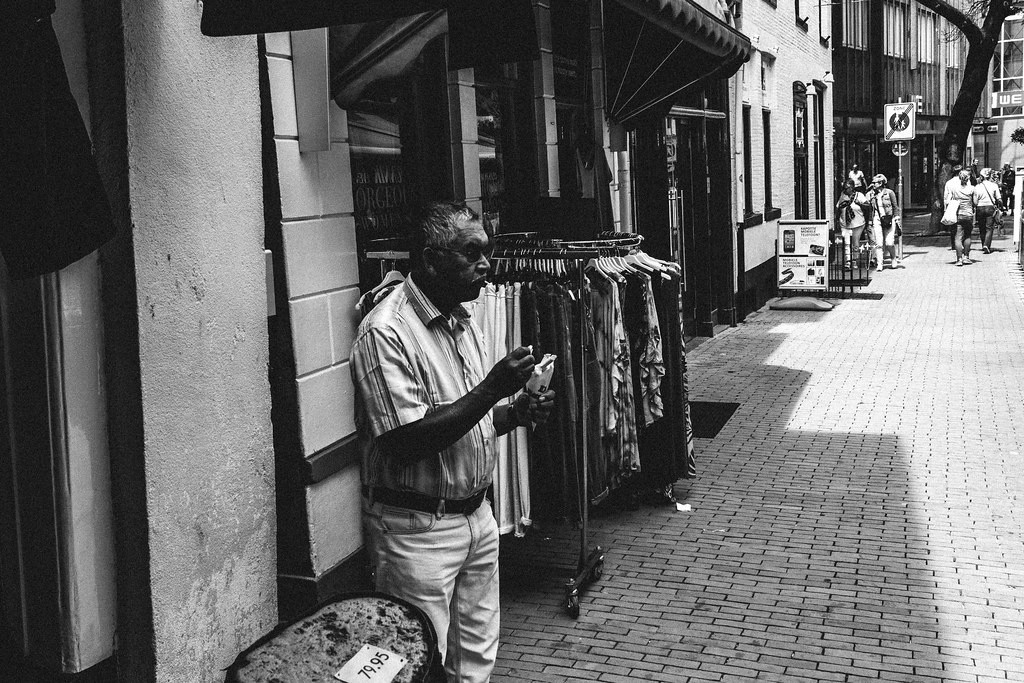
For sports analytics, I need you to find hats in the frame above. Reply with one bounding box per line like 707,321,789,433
870,174,887,183
979,167,992,179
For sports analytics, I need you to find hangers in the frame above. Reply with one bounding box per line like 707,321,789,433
483,233,683,301
353,250,407,311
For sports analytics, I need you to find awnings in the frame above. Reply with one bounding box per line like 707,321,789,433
605,0,752,128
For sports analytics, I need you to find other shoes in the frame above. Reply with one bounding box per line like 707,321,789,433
982,245,991,254
844,261,850,271
954,258,963,266
852,258,858,271
870,259,876,267
889,259,898,269
963,255,973,266
877,264,883,271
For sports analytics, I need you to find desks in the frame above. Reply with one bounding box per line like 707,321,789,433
226,592,448,683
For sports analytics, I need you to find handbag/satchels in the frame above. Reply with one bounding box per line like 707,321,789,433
880,215,892,229
940,180,961,225
992,209,1003,225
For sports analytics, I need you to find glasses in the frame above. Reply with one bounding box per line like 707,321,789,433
426,242,493,262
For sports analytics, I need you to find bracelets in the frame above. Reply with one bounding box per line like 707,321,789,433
506,401,522,426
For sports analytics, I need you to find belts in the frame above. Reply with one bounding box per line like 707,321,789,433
359,485,486,513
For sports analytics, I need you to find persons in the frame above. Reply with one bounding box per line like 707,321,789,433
349,198,556,683
943,162,1016,266
835,162,902,272
